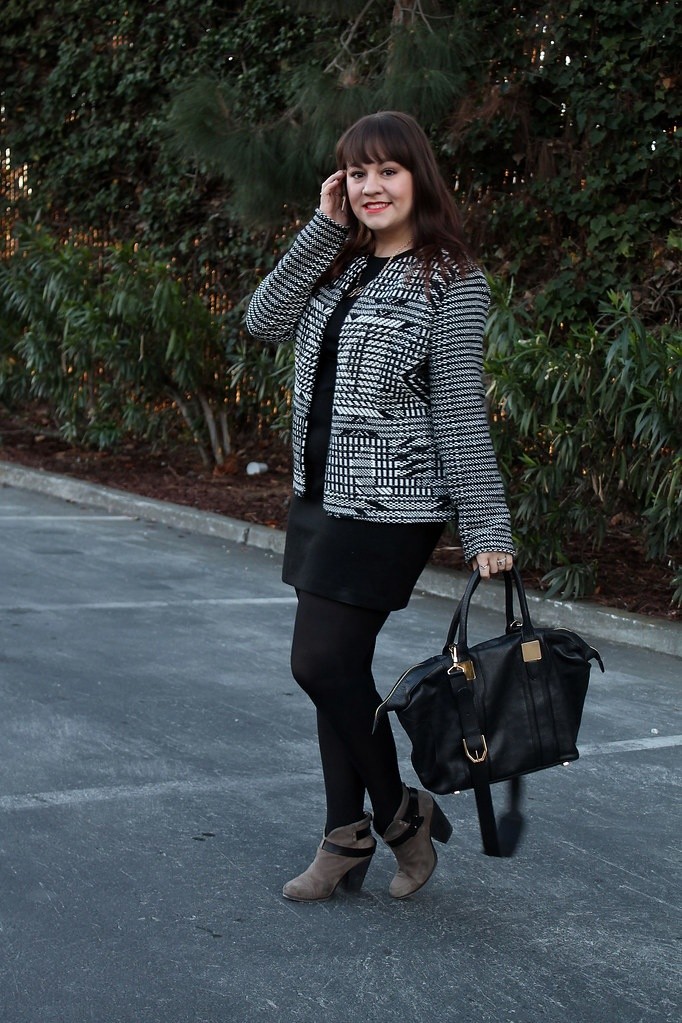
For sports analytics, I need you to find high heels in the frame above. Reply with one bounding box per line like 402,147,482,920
372,781,453,899
282,813,377,904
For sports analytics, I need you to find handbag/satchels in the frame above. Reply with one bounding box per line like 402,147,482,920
369,559,606,859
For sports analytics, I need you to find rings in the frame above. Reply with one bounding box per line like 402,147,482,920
497,557,506,566
479,564,489,570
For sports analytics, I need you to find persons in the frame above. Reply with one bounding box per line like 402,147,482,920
244,111,514,903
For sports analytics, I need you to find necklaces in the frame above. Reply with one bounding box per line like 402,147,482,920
346,237,414,297
